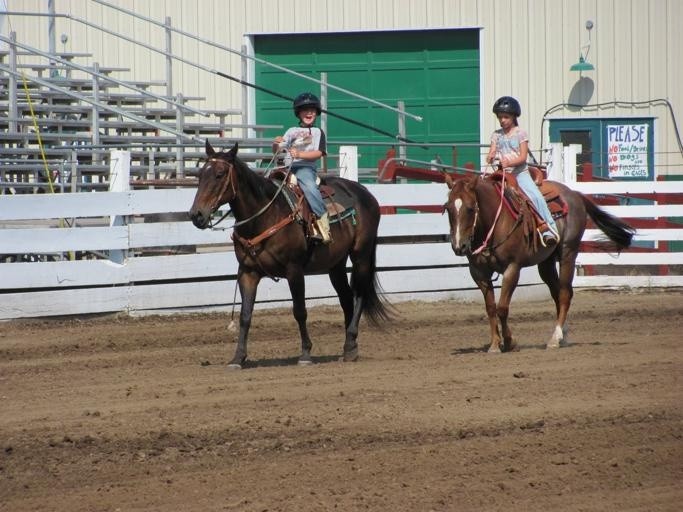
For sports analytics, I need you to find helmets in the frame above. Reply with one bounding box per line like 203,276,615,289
493,96,521,117
293,93,321,117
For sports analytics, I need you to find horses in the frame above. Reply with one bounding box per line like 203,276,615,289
442,167,637,354
188,137,402,369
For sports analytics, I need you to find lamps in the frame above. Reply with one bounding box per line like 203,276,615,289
570,20,595,71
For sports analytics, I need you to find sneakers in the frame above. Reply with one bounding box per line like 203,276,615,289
545,237,556,248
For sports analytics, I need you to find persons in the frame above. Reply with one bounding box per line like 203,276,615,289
270,93,334,246
485,96,560,248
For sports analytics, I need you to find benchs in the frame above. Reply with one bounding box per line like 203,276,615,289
0,48,404,193
